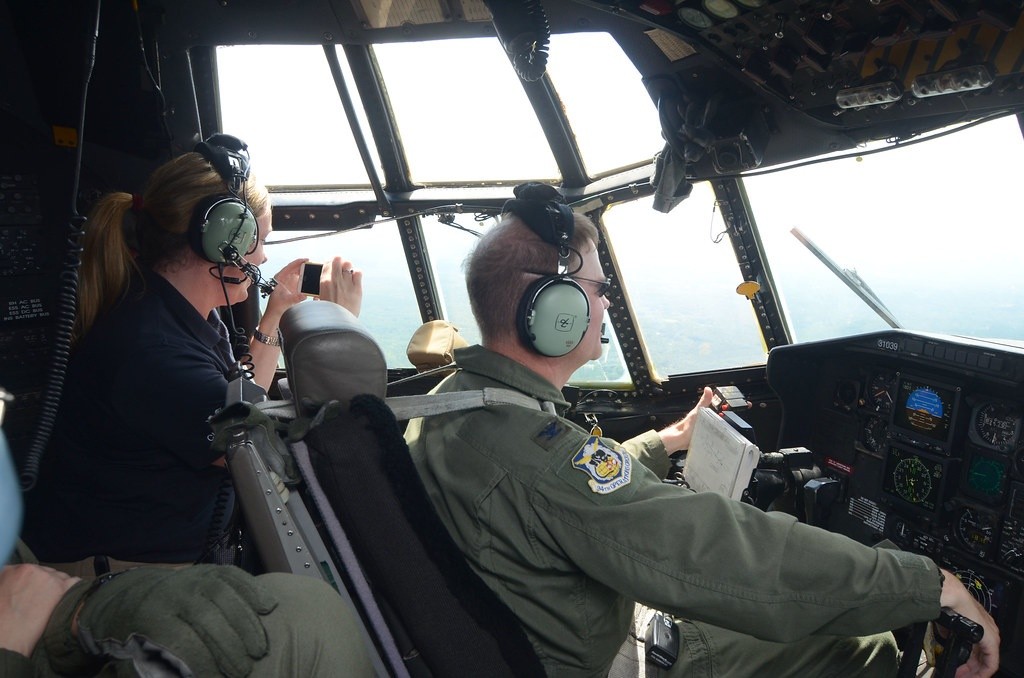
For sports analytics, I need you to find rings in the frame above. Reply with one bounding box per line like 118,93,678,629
342,269,353,274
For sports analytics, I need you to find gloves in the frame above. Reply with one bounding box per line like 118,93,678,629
27,555,280,677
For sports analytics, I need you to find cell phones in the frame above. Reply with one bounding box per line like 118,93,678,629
297,263,323,296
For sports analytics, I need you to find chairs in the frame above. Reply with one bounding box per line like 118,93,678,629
208,300,551,678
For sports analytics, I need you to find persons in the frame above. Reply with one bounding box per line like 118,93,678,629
26,134,362,564
403,182,1001,678
0,563,378,678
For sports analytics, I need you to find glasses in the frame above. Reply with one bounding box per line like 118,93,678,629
522,267,612,297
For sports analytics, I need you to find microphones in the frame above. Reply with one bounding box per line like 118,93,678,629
233,256,278,299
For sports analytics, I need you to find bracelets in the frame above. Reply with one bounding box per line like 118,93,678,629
254,326,280,346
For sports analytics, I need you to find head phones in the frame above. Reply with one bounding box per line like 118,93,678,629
502,181,590,357
188,134,260,265
